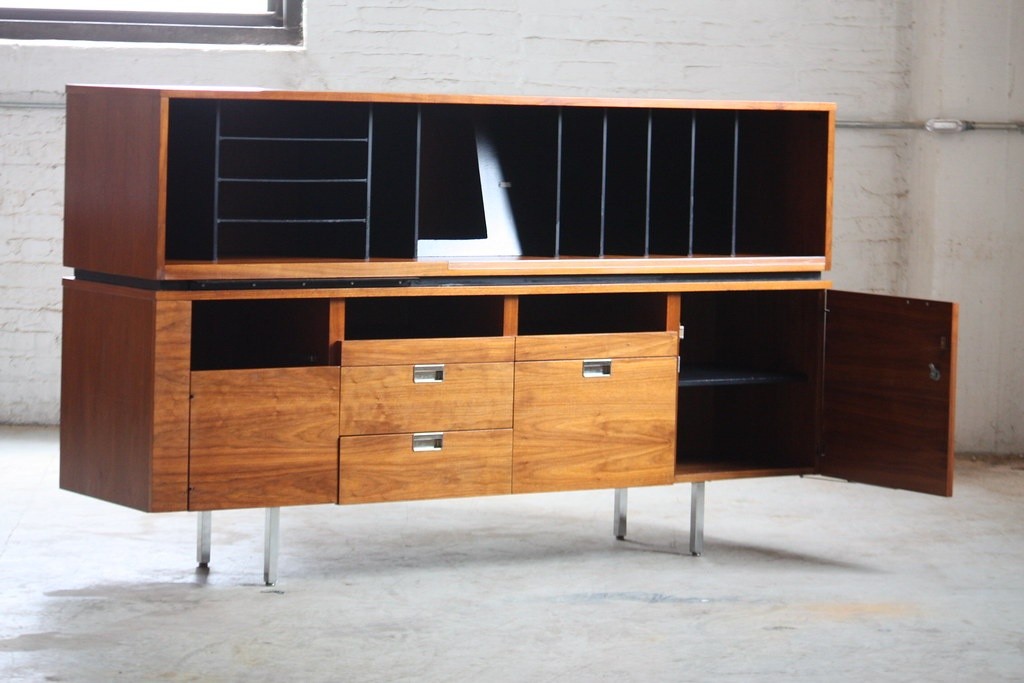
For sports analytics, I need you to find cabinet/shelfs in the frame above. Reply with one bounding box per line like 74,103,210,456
59,276,961,513
63,85,836,281
